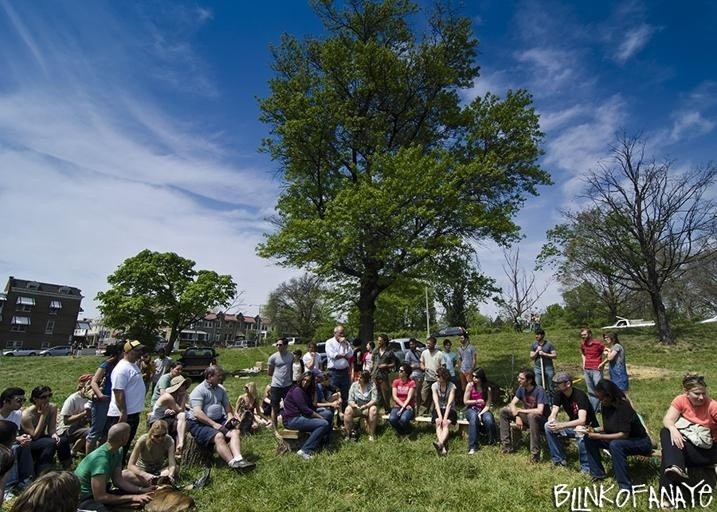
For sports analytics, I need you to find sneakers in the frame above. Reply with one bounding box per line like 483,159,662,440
231,459,256,471
467,448,475,456
663,464,690,483
70,451,84,458
194,467,211,489
501,445,513,454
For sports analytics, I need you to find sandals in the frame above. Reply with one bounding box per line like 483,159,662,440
432,441,443,453
438,446,447,455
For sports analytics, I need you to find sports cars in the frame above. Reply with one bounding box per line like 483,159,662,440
2,347,39,357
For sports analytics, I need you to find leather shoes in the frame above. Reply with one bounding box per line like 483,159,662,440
590,476,607,483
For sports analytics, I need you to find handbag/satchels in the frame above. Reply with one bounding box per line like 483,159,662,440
135,486,197,512
77,375,106,402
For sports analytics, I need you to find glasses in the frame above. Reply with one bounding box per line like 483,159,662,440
398,369,404,373
36,393,52,400
181,382,187,386
434,374,441,378
471,375,479,379
10,397,25,403
150,432,166,438
516,375,525,380
275,343,282,347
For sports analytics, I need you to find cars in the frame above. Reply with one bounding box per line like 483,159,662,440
438,326,468,336
387,337,440,373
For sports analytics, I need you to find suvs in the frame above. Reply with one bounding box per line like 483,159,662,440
226,340,255,349
271,337,301,347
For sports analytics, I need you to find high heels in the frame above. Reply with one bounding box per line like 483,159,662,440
296,449,313,459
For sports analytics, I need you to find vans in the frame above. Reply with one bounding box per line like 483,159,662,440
39,345,73,357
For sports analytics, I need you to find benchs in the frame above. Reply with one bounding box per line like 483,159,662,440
510,422,663,471
362,413,484,440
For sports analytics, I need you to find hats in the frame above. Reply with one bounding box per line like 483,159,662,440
292,350,302,355
78,372,93,382
551,371,572,383
123,339,146,353
164,374,191,393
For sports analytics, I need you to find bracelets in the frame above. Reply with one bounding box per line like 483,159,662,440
514,414,519,420
594,426,600,433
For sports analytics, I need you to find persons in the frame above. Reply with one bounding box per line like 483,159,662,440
530,329,558,388
598,332,629,393
499,368,551,463
513,313,539,333
0,325,498,512
658,373,717,499
544,371,600,473
580,379,654,493
580,329,611,412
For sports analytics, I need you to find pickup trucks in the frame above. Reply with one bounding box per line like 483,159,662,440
601,316,655,329
176,346,220,379
